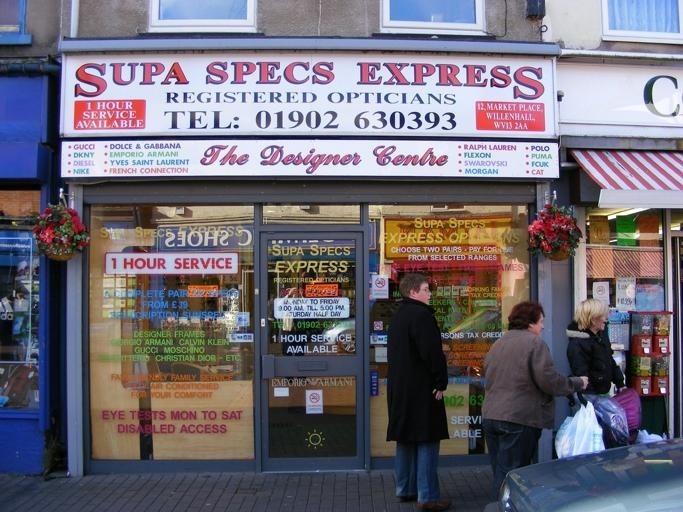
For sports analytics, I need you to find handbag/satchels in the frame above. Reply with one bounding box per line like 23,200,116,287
611,389,641,430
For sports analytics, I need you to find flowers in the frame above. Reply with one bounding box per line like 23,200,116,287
27,202,93,255
527,203,585,259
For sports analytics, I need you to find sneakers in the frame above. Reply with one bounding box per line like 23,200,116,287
416,501,451,512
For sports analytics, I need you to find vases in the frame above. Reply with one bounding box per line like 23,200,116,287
43,252,77,262
545,248,569,262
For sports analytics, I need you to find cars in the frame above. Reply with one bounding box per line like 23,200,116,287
485,438,682,512
324,320,355,353
447,306,500,343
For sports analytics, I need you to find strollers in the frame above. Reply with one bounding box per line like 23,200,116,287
568,388,643,450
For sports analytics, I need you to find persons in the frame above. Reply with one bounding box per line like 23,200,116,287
0,270,35,379
385,273,449,511
283,270,317,355
482,300,592,501
566,297,628,414
